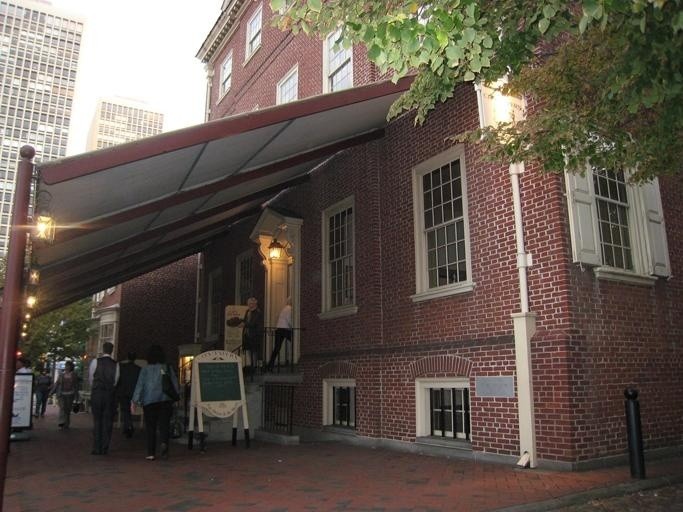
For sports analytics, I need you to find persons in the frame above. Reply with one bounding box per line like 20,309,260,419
84,341,121,455
235,296,264,369
268,296,293,370
116,350,142,435
129,345,180,461
32,368,52,418
47,361,79,429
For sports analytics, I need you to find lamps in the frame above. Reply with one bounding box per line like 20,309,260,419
268,238,285,259
29,189,56,246
28,257,39,291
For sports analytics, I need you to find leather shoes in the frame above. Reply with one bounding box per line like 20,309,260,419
144,456,155,460
91,449,101,455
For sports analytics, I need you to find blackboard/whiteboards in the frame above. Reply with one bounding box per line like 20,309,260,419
11,373,35,429
194,349,246,419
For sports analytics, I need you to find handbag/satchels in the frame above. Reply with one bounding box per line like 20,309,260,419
162,362,179,400
72,400,80,413
56,375,63,397
169,404,180,438
